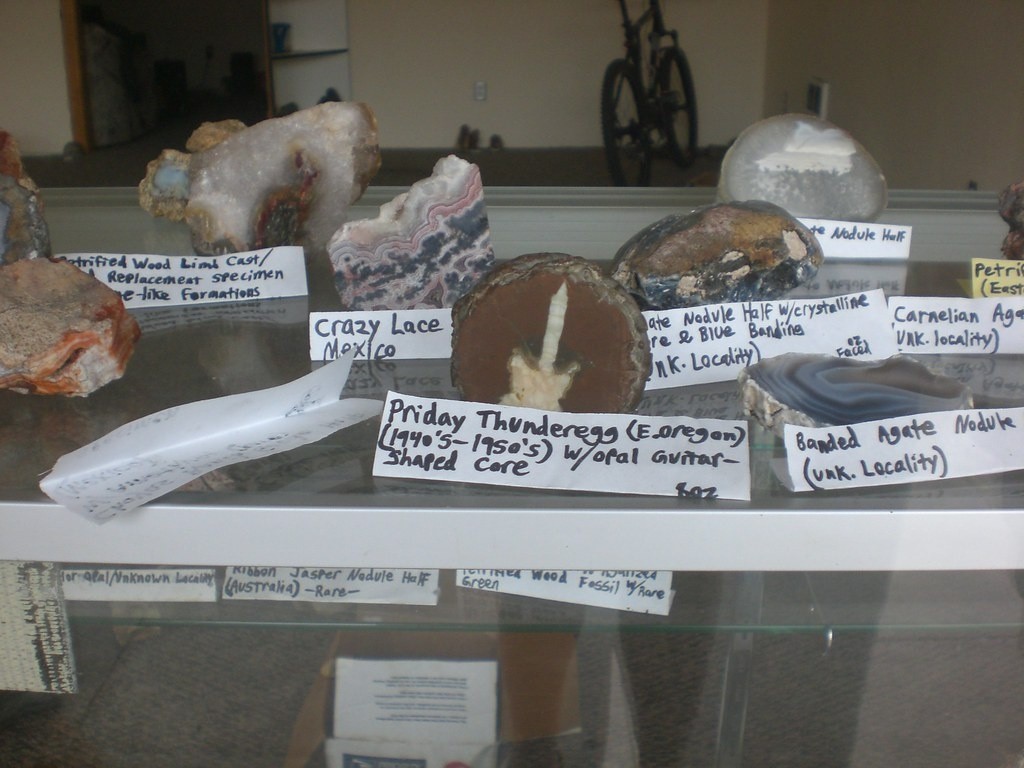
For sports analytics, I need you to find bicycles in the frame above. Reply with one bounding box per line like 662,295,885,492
601,0,700,186
